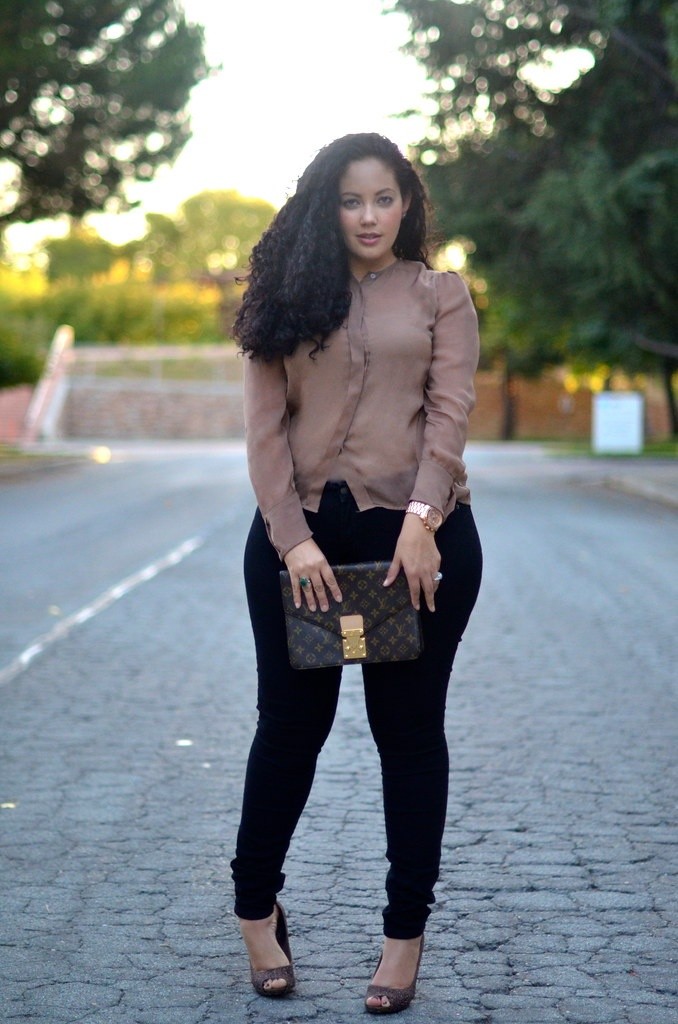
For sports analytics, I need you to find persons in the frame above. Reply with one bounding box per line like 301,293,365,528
229,133,483,1011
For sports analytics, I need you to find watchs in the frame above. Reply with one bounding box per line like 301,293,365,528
406,501,443,531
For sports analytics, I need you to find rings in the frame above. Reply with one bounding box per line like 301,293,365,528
300,578,311,587
433,573,442,580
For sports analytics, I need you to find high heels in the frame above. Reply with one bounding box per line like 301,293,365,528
249,901,295,997
364,934,424,1014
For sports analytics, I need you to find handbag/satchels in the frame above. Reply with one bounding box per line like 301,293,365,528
279,562,421,669
